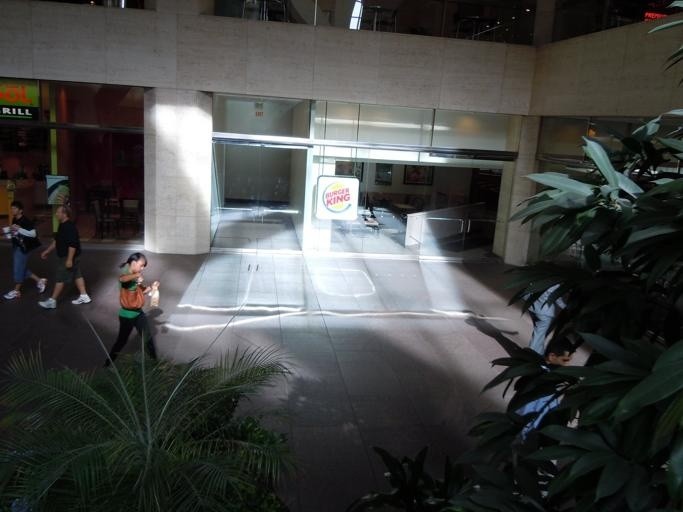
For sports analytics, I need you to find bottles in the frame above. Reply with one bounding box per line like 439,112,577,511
150,281,160,308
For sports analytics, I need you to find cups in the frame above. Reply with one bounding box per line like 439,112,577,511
2,226,12,239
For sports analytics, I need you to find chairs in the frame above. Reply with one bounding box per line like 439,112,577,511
92,197,143,240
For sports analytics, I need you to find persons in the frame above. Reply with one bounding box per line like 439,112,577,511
515,335,575,495
37,205,91,308
518,282,566,359
2,200,46,300
103,252,159,367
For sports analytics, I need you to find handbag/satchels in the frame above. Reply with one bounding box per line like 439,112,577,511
119,286,144,309
18,235,42,254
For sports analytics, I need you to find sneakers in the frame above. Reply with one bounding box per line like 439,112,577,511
38,298,56,309
3,290,21,299
71,294,91,304
37,278,49,293
